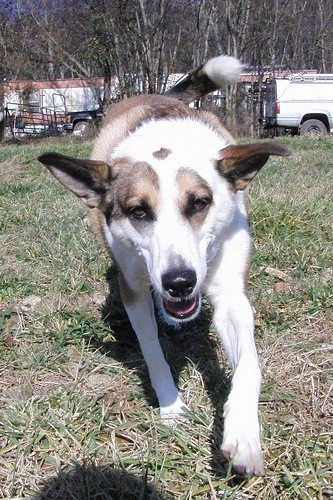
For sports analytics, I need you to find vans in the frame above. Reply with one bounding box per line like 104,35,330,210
261,73,333,139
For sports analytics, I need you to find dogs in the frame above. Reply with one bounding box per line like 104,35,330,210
37,55,292,479
